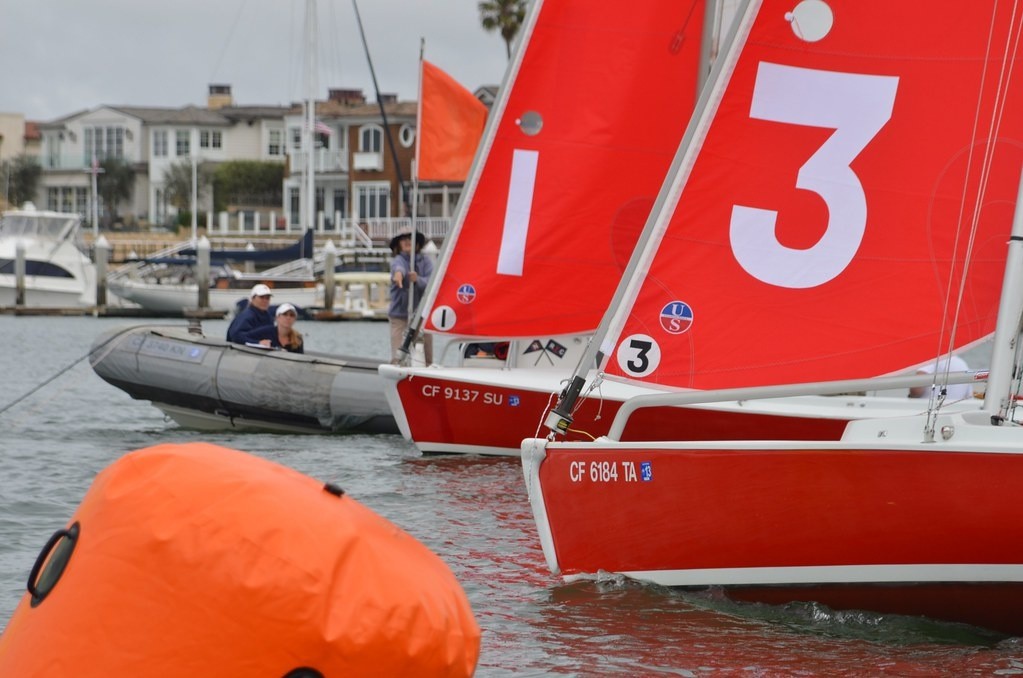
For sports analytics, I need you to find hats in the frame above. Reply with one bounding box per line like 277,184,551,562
251,284,272,299
276,303,298,320
389,226,425,255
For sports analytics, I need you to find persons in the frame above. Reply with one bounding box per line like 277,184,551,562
388,227,431,367
226,285,306,354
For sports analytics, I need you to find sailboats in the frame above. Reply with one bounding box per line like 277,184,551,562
0,0,1020,647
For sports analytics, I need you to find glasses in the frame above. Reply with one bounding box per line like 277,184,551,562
260,296,270,300
283,312,295,317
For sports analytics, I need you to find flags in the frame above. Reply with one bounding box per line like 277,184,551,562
415,59,489,184
